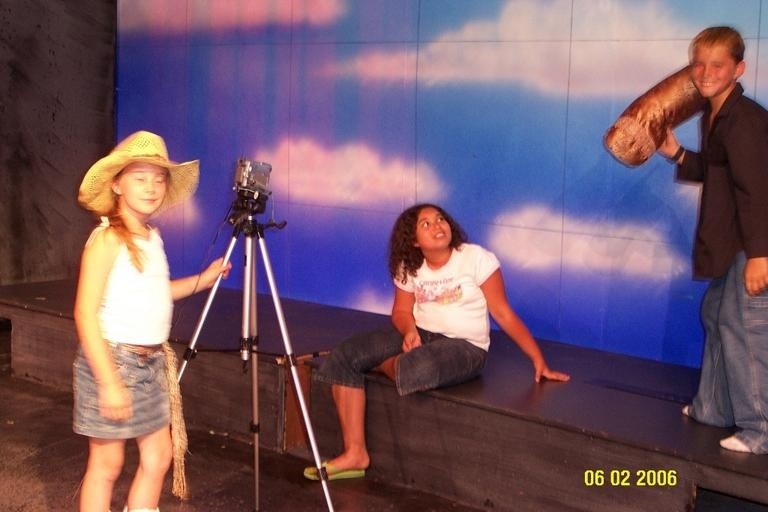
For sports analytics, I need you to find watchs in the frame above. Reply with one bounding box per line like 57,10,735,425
666,146,685,165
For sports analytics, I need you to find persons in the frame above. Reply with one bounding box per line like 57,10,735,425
655,26,768,455
72,130,232,512
301,202,571,481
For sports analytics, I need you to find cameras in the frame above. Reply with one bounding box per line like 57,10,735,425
234,157,272,193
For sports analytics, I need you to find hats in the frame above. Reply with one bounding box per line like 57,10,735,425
77,130,202,219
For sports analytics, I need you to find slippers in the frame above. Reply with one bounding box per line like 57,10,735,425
303,460,365,481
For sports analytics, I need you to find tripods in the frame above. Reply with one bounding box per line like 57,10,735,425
177,216,335,512
